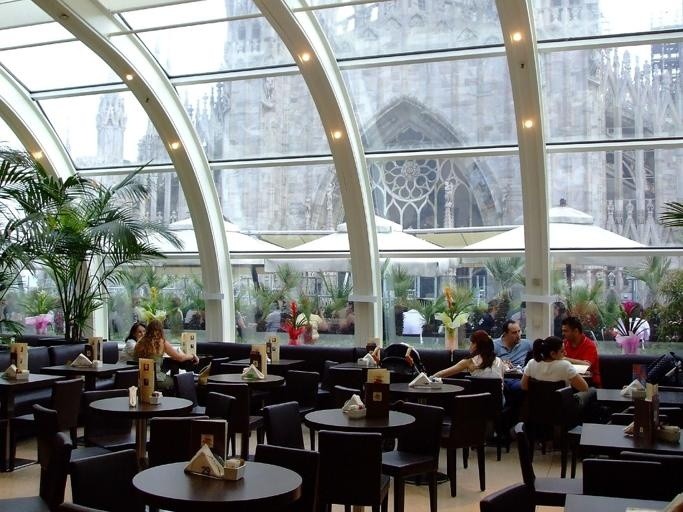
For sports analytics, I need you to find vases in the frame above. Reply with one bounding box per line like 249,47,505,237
287,326,304,345
445,326,459,349
34,316,48,336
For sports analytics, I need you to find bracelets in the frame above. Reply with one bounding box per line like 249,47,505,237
191,354,195,362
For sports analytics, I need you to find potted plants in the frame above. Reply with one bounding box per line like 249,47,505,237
17,286,62,335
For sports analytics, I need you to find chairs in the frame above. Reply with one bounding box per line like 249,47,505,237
316,429,391,511
481,482,566,512
60,449,148,511
253,443,321,511
0,334,683,477
381,400,443,511
580,455,662,499
0,431,73,512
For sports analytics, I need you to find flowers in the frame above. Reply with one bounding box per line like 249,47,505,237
612,299,648,336
433,286,474,328
283,302,309,328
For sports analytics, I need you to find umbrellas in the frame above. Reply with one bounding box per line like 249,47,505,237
128,215,282,264
264,215,449,297
463,198,647,311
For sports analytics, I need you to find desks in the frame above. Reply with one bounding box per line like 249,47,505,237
132,458,304,512
564,493,673,511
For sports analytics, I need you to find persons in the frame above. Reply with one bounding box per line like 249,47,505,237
133,295,355,340
115,322,147,379
402,300,651,452
134,320,200,390
